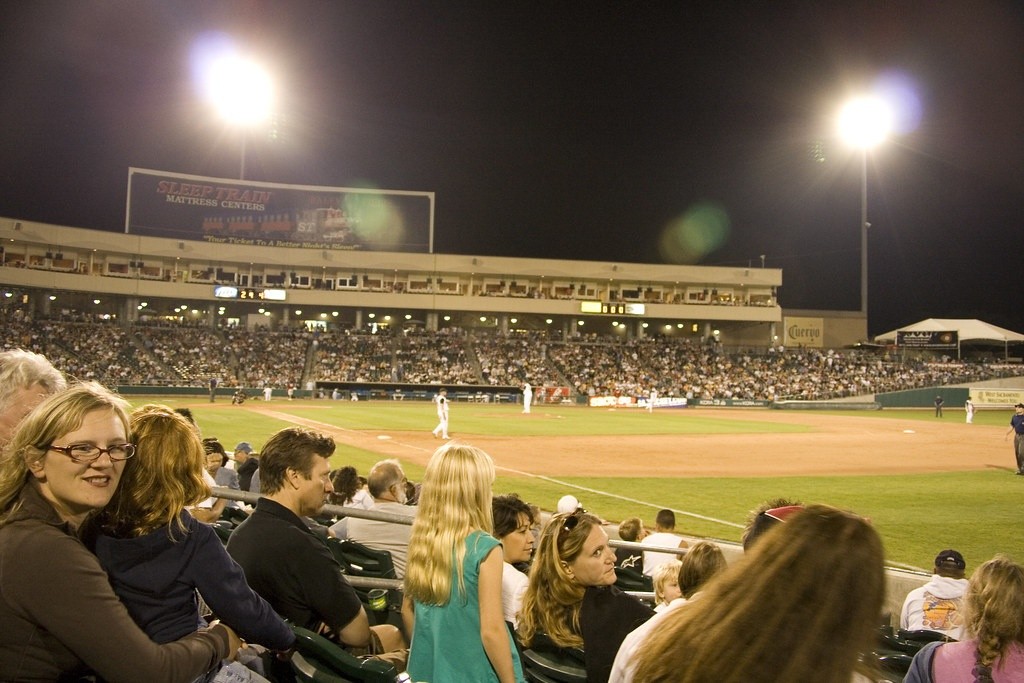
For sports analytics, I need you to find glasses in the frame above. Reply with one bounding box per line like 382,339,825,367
39,444,137,460
558,508,586,544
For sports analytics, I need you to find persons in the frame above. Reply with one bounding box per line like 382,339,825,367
934,395,946,419
225,426,375,658
901,552,1024,683
0,349,70,461
521,379,532,415
403,440,528,683
1003,402,1024,475
430,387,452,441
900,549,974,641
607,504,902,683
964,396,979,423
1,299,1024,682
82,403,298,683
515,509,658,683
0,378,249,683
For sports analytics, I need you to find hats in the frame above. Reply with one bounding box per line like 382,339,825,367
236,443,252,453
1015,403,1024,408
935,549,966,571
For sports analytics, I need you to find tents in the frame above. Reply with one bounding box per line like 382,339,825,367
874,317,1024,364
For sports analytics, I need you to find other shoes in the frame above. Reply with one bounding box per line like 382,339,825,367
432,431,438,438
442,436,450,440
1014,471,1024,475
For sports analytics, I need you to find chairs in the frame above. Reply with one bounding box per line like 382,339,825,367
213,505,958,683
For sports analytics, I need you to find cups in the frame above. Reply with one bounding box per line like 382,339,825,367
367,589,390,613
